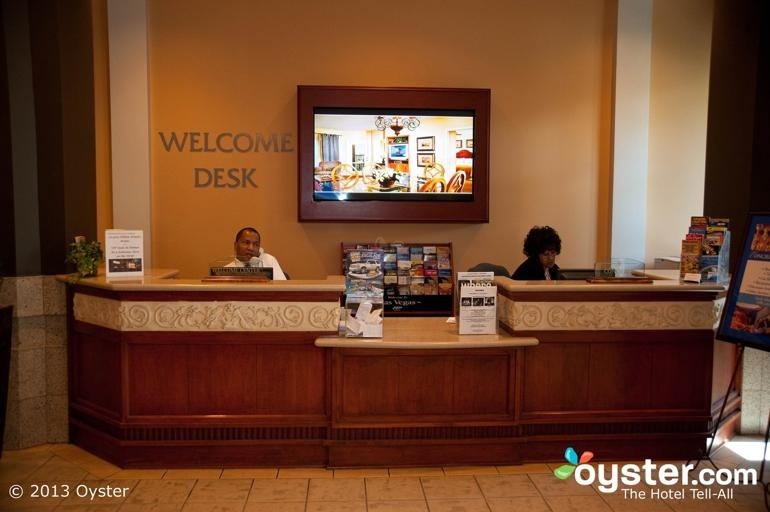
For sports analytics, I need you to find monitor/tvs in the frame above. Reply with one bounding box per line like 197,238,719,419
556,269,615,281
210,267,273,280
313,107,473,202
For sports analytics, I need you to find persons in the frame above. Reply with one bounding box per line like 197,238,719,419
512,225,568,280
224,227,287,281
392,148,405,157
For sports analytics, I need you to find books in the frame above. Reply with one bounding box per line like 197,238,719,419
680,215,730,282
343,243,453,299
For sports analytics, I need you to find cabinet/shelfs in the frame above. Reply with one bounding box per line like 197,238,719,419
341,240,456,316
